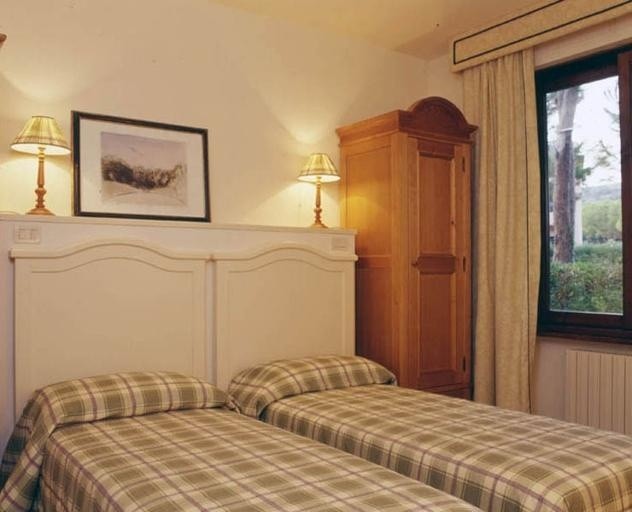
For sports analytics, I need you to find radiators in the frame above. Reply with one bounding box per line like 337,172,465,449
562,350,632,436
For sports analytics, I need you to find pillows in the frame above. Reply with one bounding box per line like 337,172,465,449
38,371,228,426
233,357,396,403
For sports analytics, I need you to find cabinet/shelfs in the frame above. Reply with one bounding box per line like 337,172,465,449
336,96,477,397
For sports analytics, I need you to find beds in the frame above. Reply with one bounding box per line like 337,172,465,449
16,371,480,512
231,357,632,510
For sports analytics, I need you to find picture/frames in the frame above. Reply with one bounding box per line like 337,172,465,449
70,111,211,221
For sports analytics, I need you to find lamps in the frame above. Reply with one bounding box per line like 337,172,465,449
11,115,71,216
298,153,341,228
